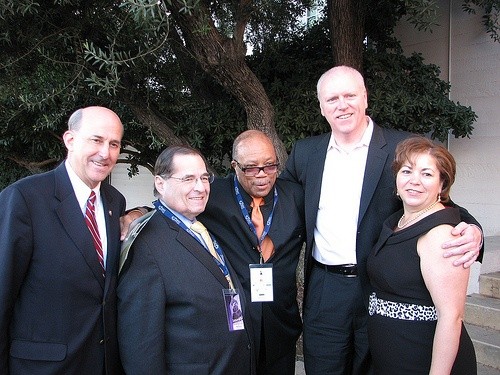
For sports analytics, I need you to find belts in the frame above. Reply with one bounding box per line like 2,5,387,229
367,292,438,321
311,257,358,277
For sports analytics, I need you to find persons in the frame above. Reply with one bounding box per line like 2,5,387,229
365,137,479,375
103,146,258,375
277,65,484,375
118,130,304,375
0,105,126,375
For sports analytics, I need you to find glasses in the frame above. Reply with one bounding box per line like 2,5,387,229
160,172,214,185
233,156,280,176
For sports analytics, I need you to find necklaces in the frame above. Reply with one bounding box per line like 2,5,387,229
397,200,440,229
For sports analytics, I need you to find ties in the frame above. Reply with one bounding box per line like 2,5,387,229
249,195,275,264
190,220,236,295
84,191,105,281
